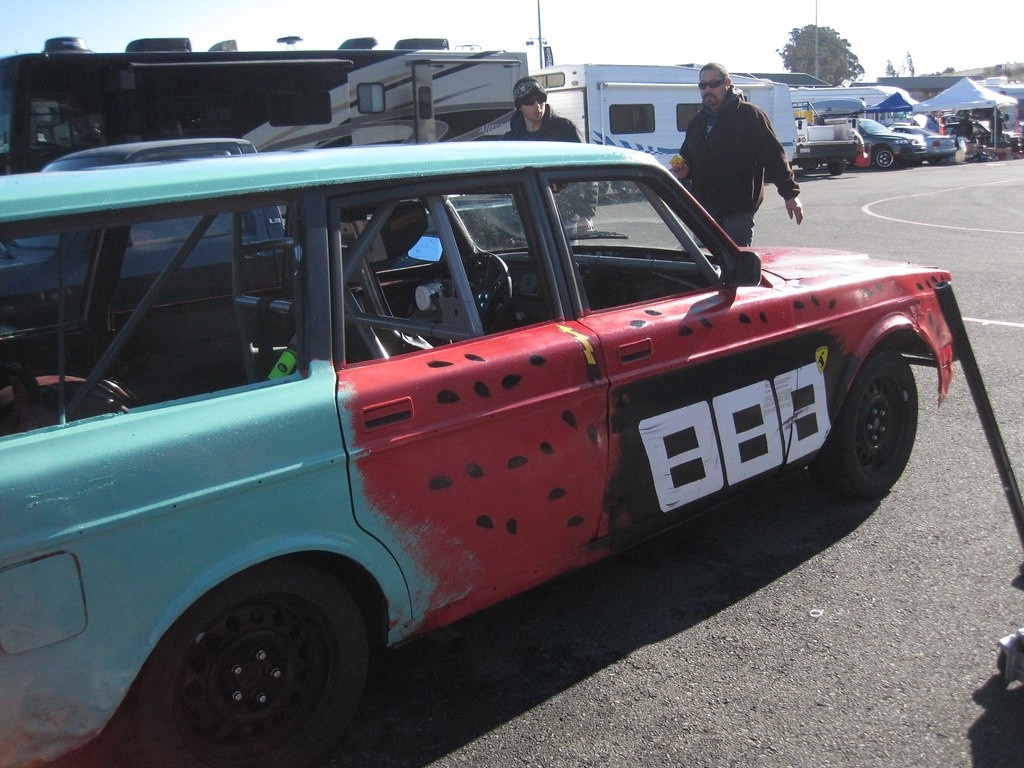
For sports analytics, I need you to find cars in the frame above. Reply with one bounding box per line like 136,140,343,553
0,141,959,768
938,114,1024,158
891,123,957,167
852,120,921,174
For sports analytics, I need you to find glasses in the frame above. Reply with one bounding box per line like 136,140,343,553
519,94,546,106
699,80,724,90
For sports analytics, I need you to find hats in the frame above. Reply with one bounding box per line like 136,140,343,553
512,76,547,105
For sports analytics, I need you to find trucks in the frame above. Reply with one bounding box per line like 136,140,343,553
789,101,857,178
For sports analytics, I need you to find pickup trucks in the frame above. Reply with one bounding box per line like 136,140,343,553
0,134,581,377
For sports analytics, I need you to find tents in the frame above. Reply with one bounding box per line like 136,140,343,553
911,77,1018,112
860,92,912,113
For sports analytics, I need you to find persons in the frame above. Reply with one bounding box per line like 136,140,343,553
668,63,804,248
990,109,1010,144
500,77,598,246
958,114,973,138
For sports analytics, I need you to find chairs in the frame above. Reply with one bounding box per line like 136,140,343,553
379,200,455,358
